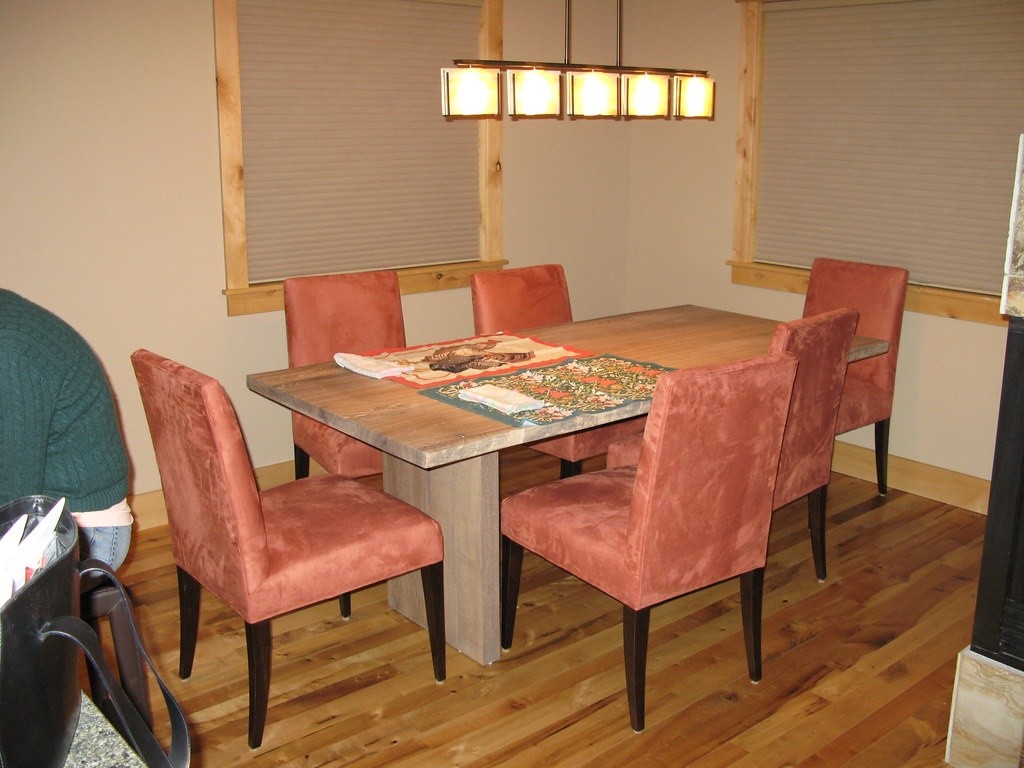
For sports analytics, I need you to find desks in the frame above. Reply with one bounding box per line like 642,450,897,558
246,304,890,667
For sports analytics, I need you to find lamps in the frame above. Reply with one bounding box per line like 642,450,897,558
440,0,716,123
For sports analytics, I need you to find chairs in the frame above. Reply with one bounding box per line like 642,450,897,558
606,304,860,583
802,257,909,532
500,351,798,733
471,264,648,479
131,349,447,751
283,270,407,481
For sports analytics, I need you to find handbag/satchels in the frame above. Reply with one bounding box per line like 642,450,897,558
0,494,193,768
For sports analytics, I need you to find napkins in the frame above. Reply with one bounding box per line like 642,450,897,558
334,353,415,379
458,383,544,416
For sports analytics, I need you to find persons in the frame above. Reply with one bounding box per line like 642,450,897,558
1,286,134,578
